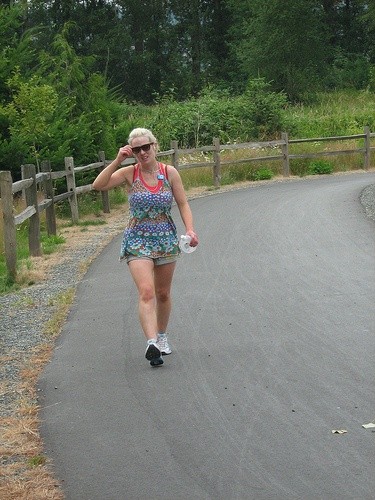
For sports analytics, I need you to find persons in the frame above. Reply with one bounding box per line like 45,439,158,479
93,128,199,366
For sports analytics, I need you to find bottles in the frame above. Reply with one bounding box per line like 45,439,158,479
180,234,197,253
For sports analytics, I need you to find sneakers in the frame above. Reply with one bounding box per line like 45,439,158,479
158,331,171,354
145,339,163,366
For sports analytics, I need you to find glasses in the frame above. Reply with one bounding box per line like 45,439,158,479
132,143,153,153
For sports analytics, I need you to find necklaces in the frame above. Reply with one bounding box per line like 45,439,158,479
141,167,161,174
